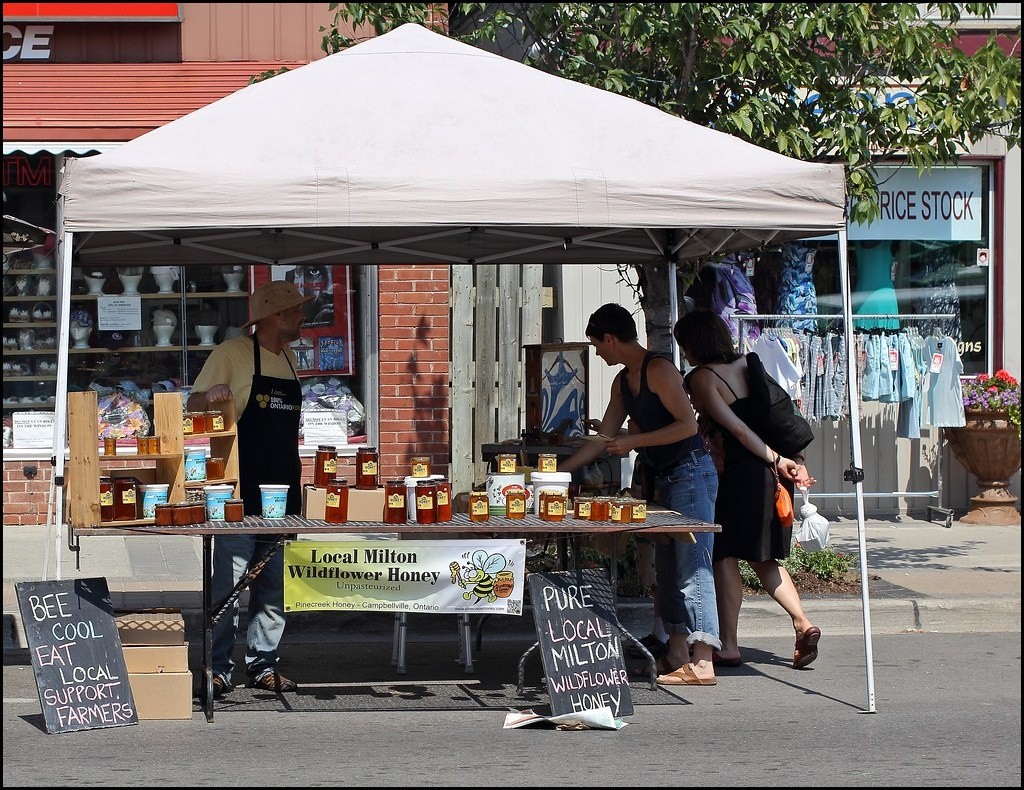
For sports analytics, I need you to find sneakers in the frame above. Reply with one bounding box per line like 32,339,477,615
202,673,224,698
253,670,297,692
625,634,671,655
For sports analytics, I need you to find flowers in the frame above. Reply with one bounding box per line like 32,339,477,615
97,389,151,439
961,369,1021,441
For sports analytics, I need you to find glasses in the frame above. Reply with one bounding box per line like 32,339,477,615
588,313,609,333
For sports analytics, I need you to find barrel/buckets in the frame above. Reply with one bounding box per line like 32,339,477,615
184,448,207,483
259,485,290,520
203,486,234,522
404,474,445,521
138,484,170,519
486,472,571,516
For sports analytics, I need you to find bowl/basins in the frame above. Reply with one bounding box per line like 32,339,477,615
119,378,180,401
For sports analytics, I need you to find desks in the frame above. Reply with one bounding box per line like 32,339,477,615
72,503,723,724
482,444,621,496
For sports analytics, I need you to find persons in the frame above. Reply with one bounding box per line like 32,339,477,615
115,265,145,294
186,279,306,688
221,300,249,341
850,239,902,331
711,250,759,342
913,241,964,346
777,244,819,332
70,310,94,349
149,264,248,294
556,303,724,686
149,309,177,347
80,264,111,295
674,311,819,672
191,301,219,346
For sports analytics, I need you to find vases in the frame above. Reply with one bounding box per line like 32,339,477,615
942,407,1022,527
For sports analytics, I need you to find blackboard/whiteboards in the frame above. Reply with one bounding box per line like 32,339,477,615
528,567,634,717
15,576,139,733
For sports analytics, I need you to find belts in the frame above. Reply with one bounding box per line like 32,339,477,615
653,447,708,480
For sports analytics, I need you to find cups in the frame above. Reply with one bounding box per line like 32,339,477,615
104,438,116,456
137,436,160,455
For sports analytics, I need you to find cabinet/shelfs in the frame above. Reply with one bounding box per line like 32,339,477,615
182,266,248,386
69,267,186,386
67,391,186,529
3,268,57,409
153,391,241,502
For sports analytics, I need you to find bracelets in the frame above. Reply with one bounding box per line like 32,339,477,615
770,455,781,466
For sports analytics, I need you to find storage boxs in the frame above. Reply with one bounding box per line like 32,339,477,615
302,483,385,522
128,670,193,720
113,606,185,646
122,642,189,674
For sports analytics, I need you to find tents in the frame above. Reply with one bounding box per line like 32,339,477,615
54,16,878,715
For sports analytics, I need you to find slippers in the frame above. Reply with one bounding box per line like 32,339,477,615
791,627,821,670
629,658,676,680
654,662,718,686
709,649,743,667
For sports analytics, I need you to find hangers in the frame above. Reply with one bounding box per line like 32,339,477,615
761,314,946,351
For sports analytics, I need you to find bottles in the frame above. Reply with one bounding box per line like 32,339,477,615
356,447,378,490
155,502,206,526
113,477,137,521
81,353,137,378
538,454,557,472
385,458,452,524
183,411,225,435
224,500,244,522
314,445,349,524
568,463,647,524
100,478,113,522
498,454,517,474
506,490,567,522
206,458,225,480
469,492,489,523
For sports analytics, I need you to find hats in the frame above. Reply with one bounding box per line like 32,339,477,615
189,308,221,326
153,309,179,326
102,327,130,351
240,279,316,330
30,233,58,256
71,310,95,327
148,265,181,276
80,266,109,280
115,265,144,277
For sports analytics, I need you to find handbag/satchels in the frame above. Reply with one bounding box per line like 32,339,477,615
767,447,793,528
744,352,815,459
795,486,830,554
